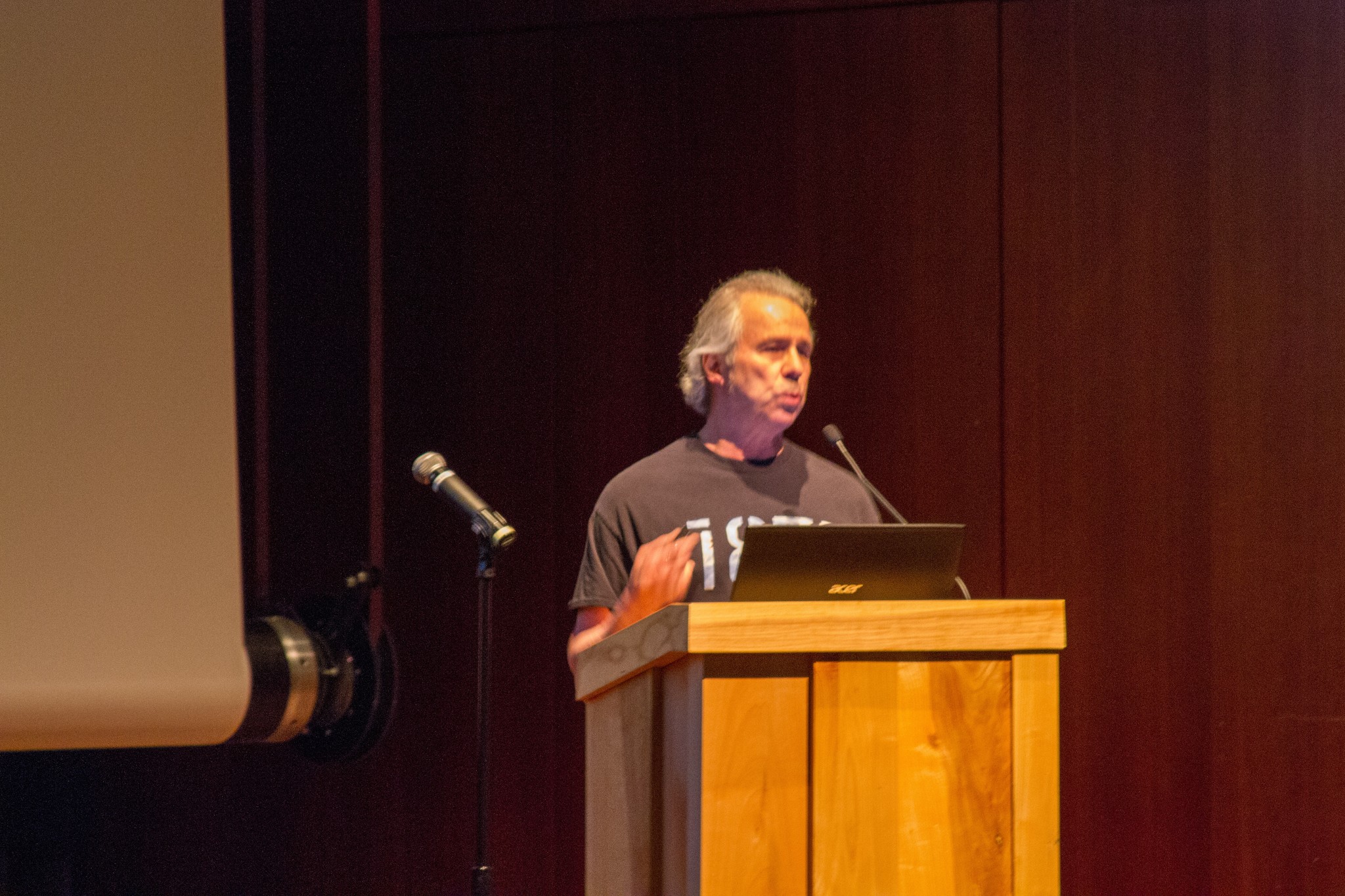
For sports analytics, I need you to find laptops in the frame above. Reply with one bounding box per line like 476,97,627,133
728,521,967,603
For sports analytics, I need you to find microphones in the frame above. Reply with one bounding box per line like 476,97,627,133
822,423,971,598
413,450,516,549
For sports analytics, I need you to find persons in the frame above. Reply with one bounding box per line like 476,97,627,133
567,265,886,674
